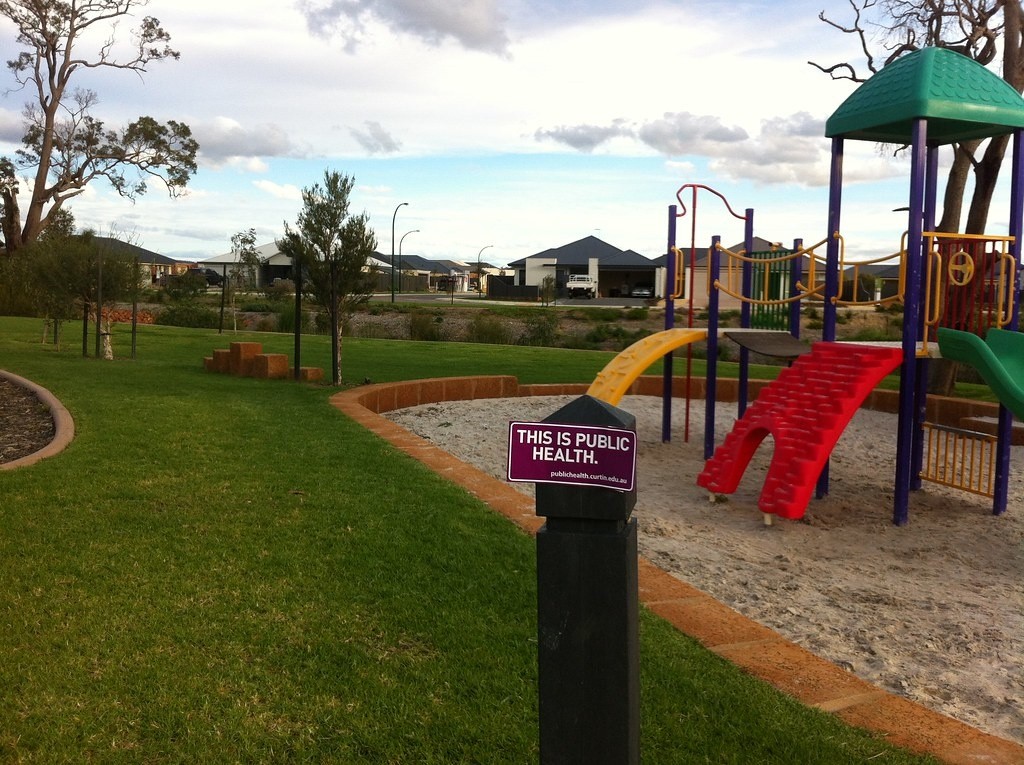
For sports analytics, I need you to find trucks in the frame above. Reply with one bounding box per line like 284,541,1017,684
565,274,598,299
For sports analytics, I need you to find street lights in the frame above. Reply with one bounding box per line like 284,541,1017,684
391,202,409,304
477,246,494,291
399,229,421,294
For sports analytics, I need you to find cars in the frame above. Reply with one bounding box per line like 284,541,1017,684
631,282,654,298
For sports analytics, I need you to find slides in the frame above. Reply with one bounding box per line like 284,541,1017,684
937,326,1024,422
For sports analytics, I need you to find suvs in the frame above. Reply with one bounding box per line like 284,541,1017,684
186,267,224,289
